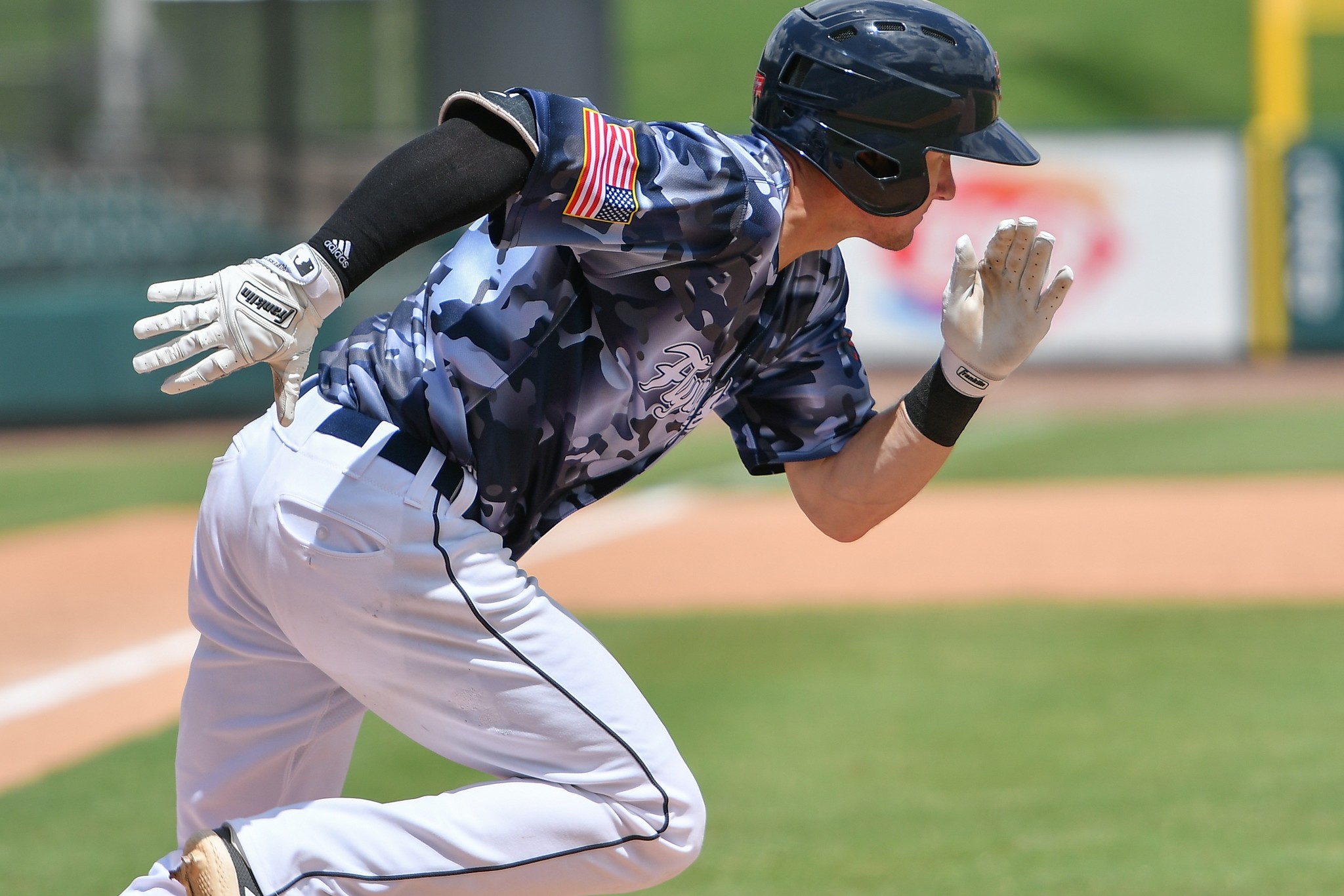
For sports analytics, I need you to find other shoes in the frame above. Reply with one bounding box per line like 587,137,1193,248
183,824,262,895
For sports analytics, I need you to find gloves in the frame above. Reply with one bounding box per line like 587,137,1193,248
941,218,1073,397
132,243,344,426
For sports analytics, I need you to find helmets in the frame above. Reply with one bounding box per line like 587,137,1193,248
750,0,1040,218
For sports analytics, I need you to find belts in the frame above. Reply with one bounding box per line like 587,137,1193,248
296,378,484,525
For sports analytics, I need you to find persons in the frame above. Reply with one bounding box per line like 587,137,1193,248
107,3,1074,895
